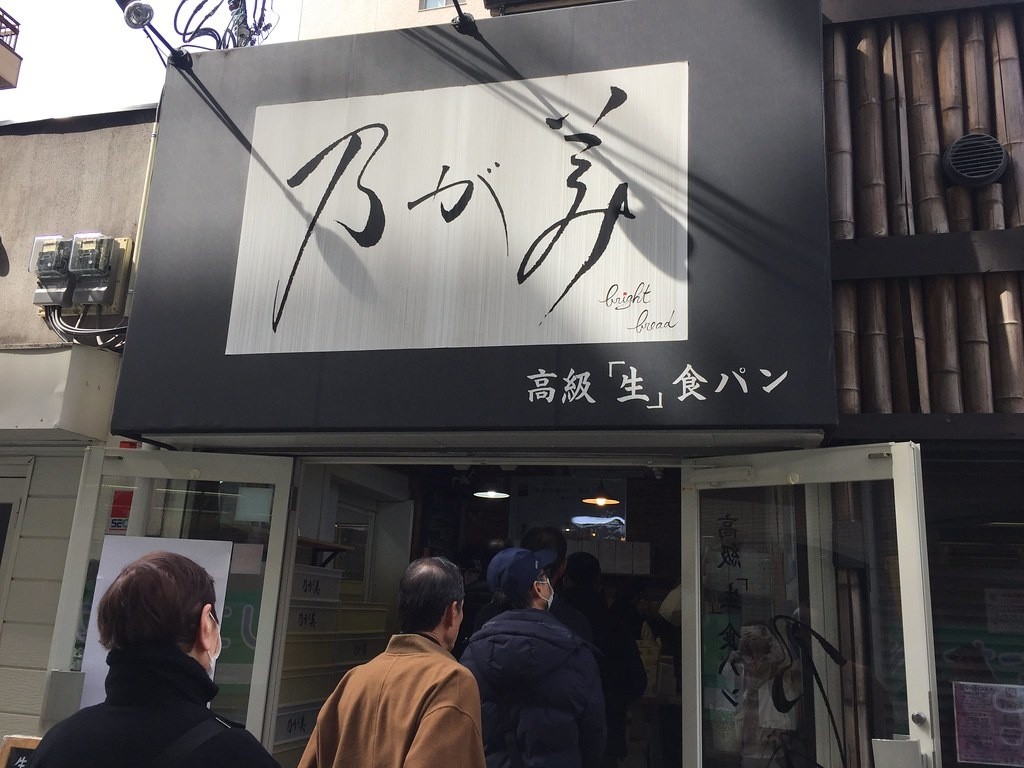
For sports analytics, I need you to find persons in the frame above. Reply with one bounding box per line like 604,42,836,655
296,557,487,768
458,546,605,768
460,525,657,768
24,551,282,768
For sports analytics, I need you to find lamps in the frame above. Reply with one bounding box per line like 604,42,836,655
581,477,620,506
116,0,189,68
470,467,512,499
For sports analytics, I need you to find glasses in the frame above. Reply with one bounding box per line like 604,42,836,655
539,569,552,579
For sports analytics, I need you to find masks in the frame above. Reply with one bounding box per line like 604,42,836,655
534,578,555,610
202,611,222,680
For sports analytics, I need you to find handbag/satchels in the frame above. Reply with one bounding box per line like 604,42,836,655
636,621,675,705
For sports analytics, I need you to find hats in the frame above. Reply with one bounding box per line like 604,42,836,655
486,548,559,593
